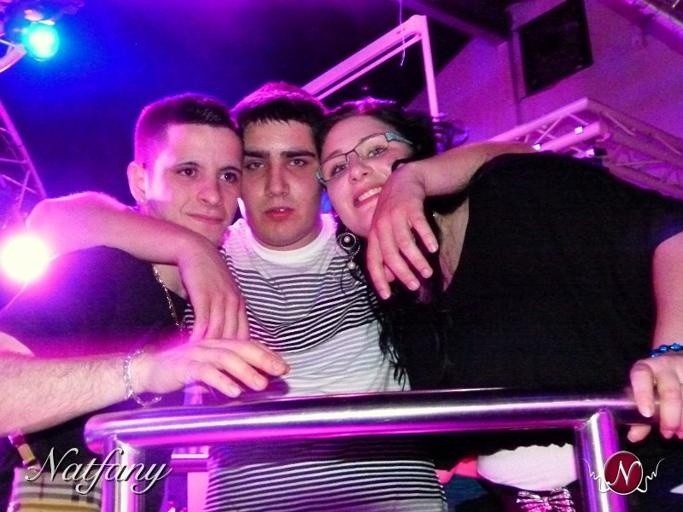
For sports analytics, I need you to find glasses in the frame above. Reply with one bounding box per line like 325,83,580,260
315,132,416,187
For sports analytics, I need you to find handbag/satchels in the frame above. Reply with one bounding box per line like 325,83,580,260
7,461,110,512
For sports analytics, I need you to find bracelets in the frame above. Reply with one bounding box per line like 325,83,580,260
644,340,682,360
120,345,166,409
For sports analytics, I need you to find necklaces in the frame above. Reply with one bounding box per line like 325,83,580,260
149,259,191,344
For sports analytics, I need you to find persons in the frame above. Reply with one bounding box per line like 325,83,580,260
312,94,682,508
21,80,539,510
0,90,292,510
